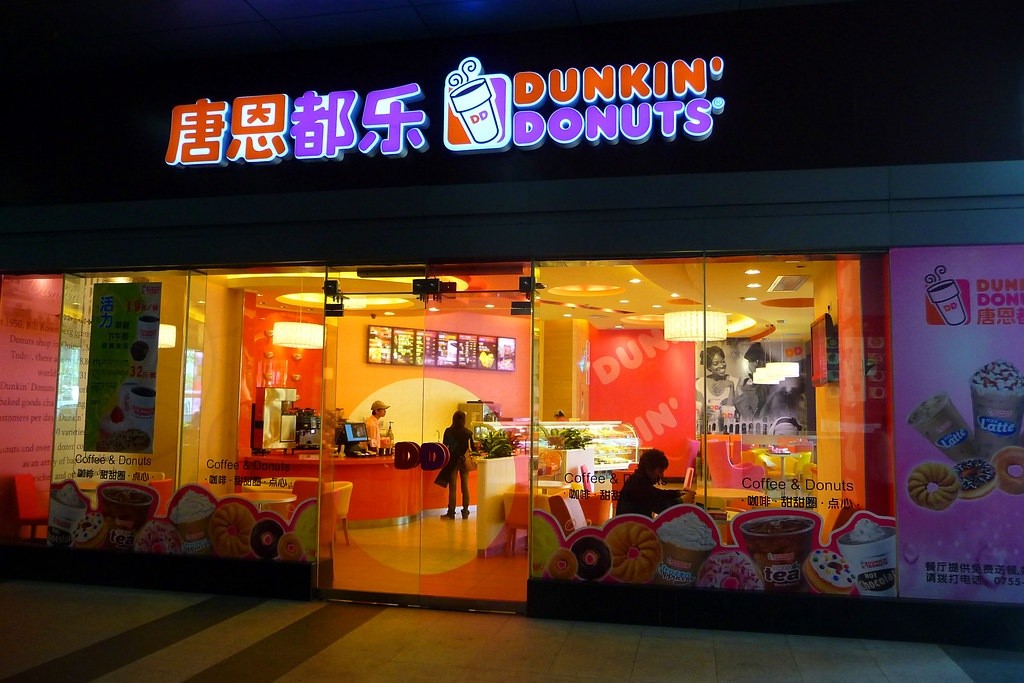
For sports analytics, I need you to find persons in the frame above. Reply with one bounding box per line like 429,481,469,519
439,410,475,519
615,448,696,518
363,399,390,447
552,407,567,422
695,346,806,422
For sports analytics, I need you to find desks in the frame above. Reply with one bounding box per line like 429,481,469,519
702,506,747,525
241,477,319,493
765,451,799,482
217,492,297,512
74,480,102,493
690,487,765,509
519,481,569,495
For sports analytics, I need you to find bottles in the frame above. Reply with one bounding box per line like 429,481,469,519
386,421,394,454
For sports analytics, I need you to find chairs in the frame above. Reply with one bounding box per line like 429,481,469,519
10,474,50,541
289,479,355,561
133,470,172,517
503,462,695,555
724,440,818,490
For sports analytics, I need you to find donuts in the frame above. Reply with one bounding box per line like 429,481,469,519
990,446,1024,496
544,521,662,583
951,458,997,499
802,549,856,595
906,463,958,511
699,548,765,589
72,502,306,561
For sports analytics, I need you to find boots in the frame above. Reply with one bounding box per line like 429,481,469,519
461,505,470,519
440,505,456,519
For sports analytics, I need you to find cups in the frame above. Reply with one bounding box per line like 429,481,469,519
137,313,158,352
119,381,156,434
169,512,214,555
907,396,976,463
48,491,87,548
96,487,152,551
972,388,1024,457
379,434,392,455
837,527,898,597
741,516,814,590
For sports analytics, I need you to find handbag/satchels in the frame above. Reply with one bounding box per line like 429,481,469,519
463,438,477,471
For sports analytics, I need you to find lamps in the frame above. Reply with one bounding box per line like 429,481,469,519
272,279,327,350
752,320,800,386
158,323,176,350
664,263,727,342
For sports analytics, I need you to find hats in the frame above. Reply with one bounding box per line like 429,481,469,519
554,409,564,417
371,401,390,410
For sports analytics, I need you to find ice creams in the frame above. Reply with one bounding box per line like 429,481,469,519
969,361,1024,459
838,516,898,597
655,511,715,586
169,489,216,555
47,485,88,548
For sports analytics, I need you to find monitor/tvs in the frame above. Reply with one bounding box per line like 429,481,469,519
811,313,838,386
335,423,368,446
